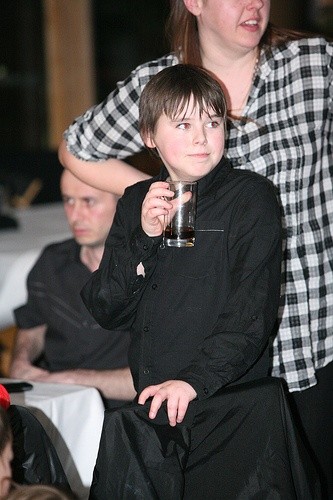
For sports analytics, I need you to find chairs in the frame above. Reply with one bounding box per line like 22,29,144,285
94,379,322,500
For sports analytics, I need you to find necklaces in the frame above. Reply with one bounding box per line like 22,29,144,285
251,57,258,83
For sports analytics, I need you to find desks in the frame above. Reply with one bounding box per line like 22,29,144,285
0,377,104,499
0,203,74,332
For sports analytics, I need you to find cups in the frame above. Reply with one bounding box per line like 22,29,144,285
162,180,196,248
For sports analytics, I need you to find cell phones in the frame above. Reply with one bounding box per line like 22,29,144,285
1,382,33,393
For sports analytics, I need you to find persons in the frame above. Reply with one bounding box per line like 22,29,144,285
7,168,138,412
0,403,67,499
79,63,288,426
58,1,332,394
